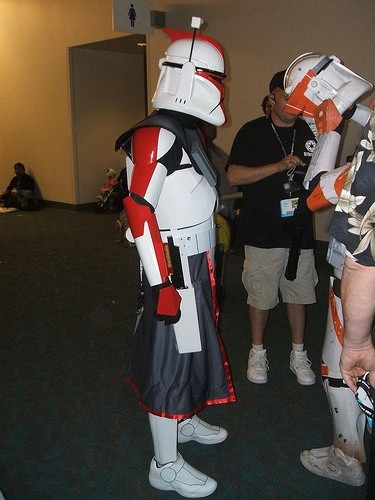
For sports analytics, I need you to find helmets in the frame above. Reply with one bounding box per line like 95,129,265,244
281,52,375,137
151,16,225,127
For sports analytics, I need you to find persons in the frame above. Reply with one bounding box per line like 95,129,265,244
282,51,375,487
0,162,37,212
115,15,236,497
225,51,375,486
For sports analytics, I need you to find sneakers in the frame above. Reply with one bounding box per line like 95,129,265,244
296,442,366,487
289,350,316,386
148,451,218,498
176,414,229,445
246,348,270,384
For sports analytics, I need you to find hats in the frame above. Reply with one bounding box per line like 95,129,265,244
269,70,287,93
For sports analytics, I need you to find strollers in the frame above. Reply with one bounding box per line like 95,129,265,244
96,168,128,216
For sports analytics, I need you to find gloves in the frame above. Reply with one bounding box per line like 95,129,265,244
151,281,182,326
